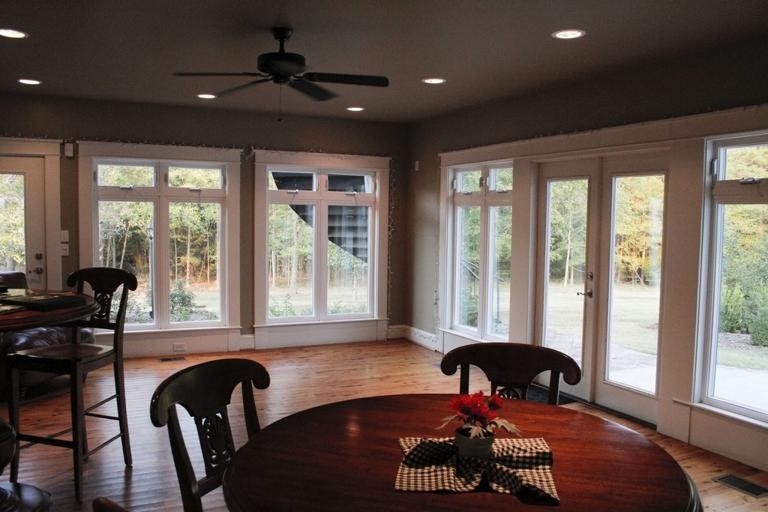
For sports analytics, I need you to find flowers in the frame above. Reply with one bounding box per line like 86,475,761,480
434,391,522,440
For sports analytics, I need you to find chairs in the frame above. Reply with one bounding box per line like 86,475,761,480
441,342,581,405
151,358,269,512
91,497,130,512
5,267,138,502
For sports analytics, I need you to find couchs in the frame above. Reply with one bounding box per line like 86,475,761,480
0,272,97,398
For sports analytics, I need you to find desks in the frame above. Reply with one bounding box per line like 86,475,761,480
222,393,703,511
0,290,101,404
0,481,50,512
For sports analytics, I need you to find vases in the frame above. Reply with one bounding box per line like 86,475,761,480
455,427,495,462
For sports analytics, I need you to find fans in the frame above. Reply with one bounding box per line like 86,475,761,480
174,26,390,101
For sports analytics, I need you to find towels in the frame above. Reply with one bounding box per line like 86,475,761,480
394,437,560,503
